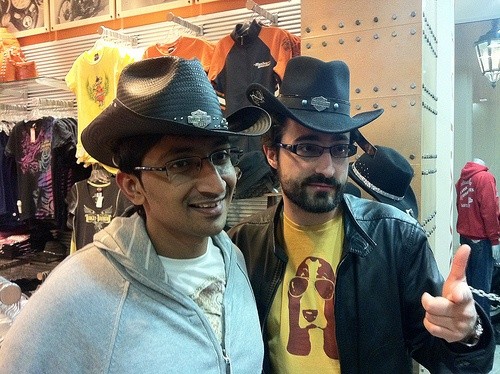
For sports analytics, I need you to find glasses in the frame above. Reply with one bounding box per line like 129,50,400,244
274,142,358,159
130,146,244,187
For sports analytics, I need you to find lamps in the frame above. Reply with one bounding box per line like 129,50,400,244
473,18,500,88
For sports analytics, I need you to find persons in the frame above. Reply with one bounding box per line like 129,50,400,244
225,57,497,374
0,56,272,374
455,158,500,316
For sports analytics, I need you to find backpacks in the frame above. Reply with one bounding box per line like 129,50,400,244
0,31,20,54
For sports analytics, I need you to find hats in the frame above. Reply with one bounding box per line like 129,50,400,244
246,55,385,134
24,241,69,263
235,150,281,199
80,55,272,169
347,144,419,220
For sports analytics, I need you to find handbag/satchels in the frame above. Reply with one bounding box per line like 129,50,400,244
15,58,38,80
0,45,26,83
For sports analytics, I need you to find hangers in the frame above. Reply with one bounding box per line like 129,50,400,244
218,4,293,41
0,96,77,135
75,163,120,188
147,16,205,54
79,25,140,60
